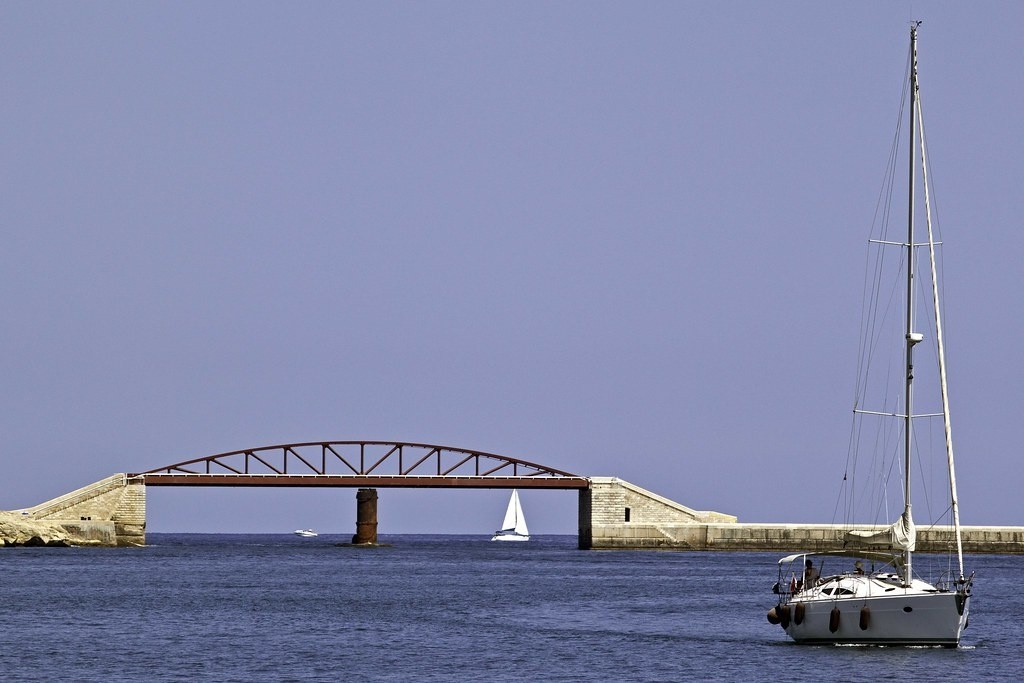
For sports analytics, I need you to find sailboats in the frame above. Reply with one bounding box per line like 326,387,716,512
763,22,977,648
490,488,531,542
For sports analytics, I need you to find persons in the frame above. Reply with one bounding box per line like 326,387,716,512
853,560,865,575
792,559,818,595
